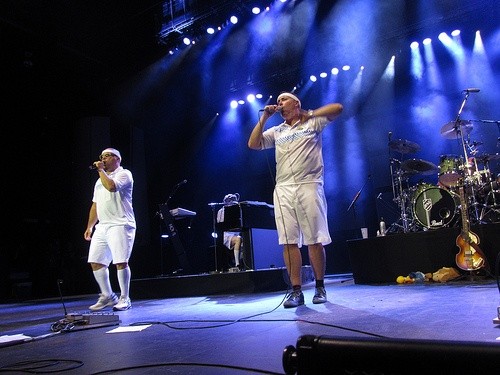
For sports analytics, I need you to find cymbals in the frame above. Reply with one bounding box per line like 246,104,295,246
399,158,437,175
440,120,473,139
388,139,417,151
472,154,500,163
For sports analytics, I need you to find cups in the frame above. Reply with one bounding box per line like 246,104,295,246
361,228,369,239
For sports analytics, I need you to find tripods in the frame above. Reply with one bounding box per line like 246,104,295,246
381,138,428,237
452,93,500,229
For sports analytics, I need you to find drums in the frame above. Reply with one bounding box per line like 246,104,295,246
473,169,491,187
413,186,461,230
439,180,472,208
437,155,464,187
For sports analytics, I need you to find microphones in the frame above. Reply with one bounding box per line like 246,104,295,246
387,132,393,138
463,88,480,93
176,180,187,186
90,161,107,169
260,106,282,112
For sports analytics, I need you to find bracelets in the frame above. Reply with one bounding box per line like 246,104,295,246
308,108,314,117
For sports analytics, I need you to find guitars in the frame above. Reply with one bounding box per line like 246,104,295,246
456,187,485,270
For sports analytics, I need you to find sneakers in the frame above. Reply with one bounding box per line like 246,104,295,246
89,292,119,310
112,296,131,311
283,289,305,308
312,287,327,303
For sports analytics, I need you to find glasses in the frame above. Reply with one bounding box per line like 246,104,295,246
99,154,115,160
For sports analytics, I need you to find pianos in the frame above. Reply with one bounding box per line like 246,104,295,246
221,202,279,272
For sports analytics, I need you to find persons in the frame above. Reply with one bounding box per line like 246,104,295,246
83,147,136,311
222,194,246,268
247,90,343,306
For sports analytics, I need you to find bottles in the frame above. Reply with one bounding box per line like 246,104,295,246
379,217,386,236
